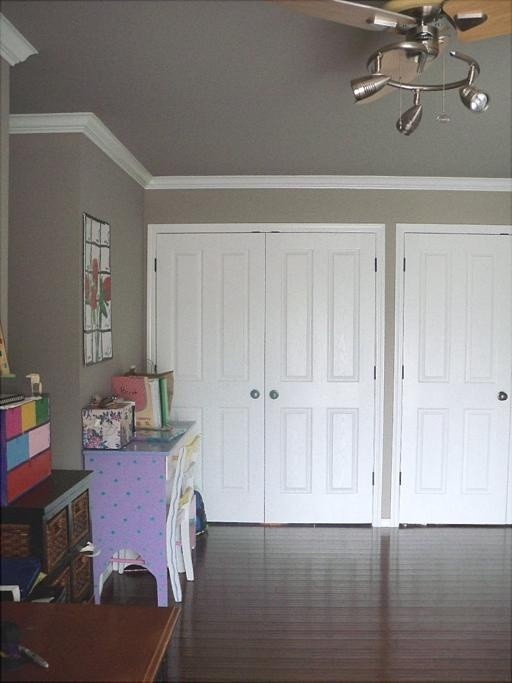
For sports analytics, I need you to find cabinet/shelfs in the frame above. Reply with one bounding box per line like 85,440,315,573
0,470,94,602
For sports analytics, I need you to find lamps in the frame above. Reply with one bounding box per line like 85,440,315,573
350,25,490,135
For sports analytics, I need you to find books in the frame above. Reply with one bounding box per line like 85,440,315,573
0,392,25,406
110,374,187,444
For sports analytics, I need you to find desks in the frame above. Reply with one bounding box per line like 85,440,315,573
0,602,182,683
82,421,196,607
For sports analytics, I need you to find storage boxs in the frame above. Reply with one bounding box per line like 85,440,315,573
82,400,136,450
0,393,52,505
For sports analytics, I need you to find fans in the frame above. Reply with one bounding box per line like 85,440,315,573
270,0,512,106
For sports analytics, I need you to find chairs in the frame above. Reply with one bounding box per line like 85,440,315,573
119,433,202,603
